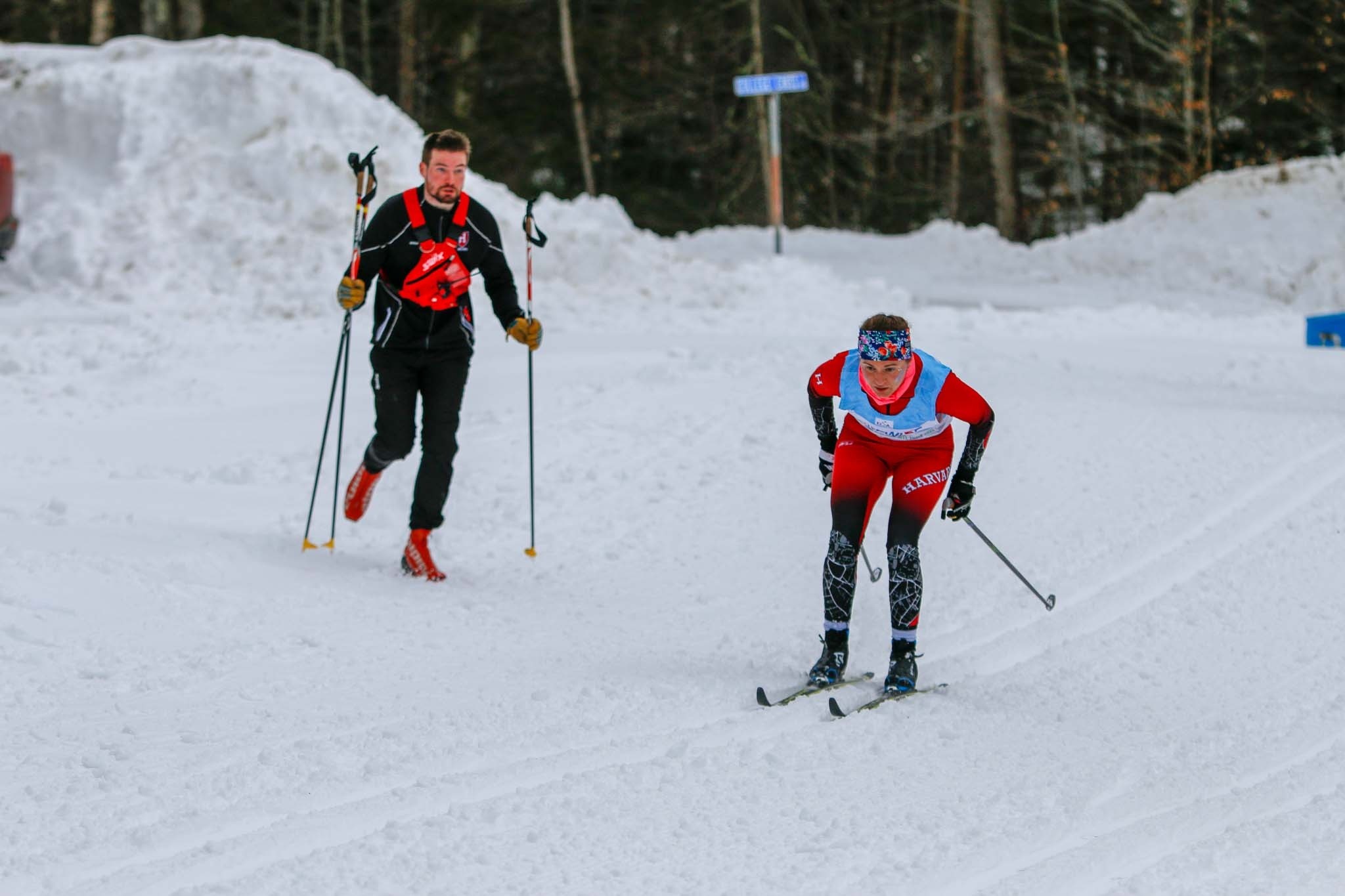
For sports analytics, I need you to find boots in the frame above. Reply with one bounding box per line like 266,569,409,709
348,460,381,521
402,528,447,581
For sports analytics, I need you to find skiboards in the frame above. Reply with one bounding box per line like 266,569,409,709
756,671,948,718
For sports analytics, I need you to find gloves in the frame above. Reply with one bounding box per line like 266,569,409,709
941,470,977,521
337,277,365,310
818,440,836,489
507,319,541,351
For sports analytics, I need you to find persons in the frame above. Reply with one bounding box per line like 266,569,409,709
807,313,995,698
337,130,543,581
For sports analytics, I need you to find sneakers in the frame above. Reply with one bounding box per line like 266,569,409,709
807,627,849,685
884,639,922,693
